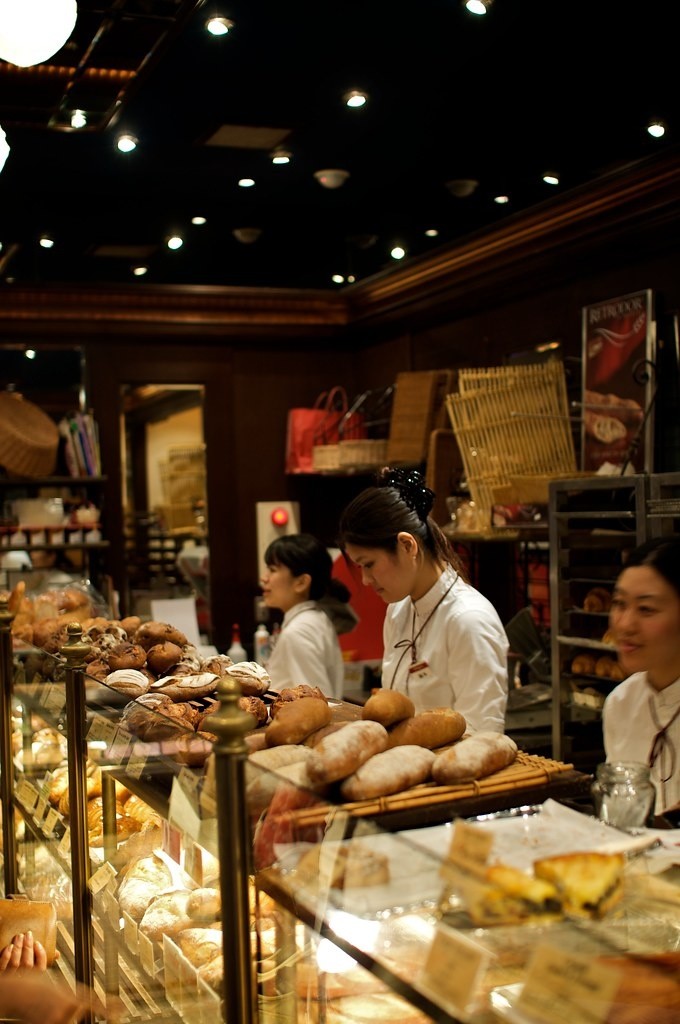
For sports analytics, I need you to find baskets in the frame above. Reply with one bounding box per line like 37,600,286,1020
0,391,60,480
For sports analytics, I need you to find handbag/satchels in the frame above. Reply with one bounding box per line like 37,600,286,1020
283,387,366,477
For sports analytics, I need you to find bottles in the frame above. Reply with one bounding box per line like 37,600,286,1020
590,762,657,830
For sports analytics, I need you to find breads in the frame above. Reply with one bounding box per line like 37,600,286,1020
217,686,519,813
569,584,628,681
0,582,331,766
11,705,435,1024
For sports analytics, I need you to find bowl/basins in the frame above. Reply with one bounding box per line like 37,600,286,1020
313,437,386,472
492,469,600,507
76,510,101,524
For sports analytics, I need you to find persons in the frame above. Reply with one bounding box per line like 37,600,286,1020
338,467,511,735
601,533,680,829
0,930,60,978
260,532,344,701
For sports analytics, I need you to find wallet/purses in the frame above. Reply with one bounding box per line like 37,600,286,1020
1,892,56,968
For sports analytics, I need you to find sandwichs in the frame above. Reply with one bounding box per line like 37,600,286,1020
438,823,627,925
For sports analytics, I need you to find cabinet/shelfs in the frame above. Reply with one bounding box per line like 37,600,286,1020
547,471,679,763
0,591,680,1024
0,471,110,634
